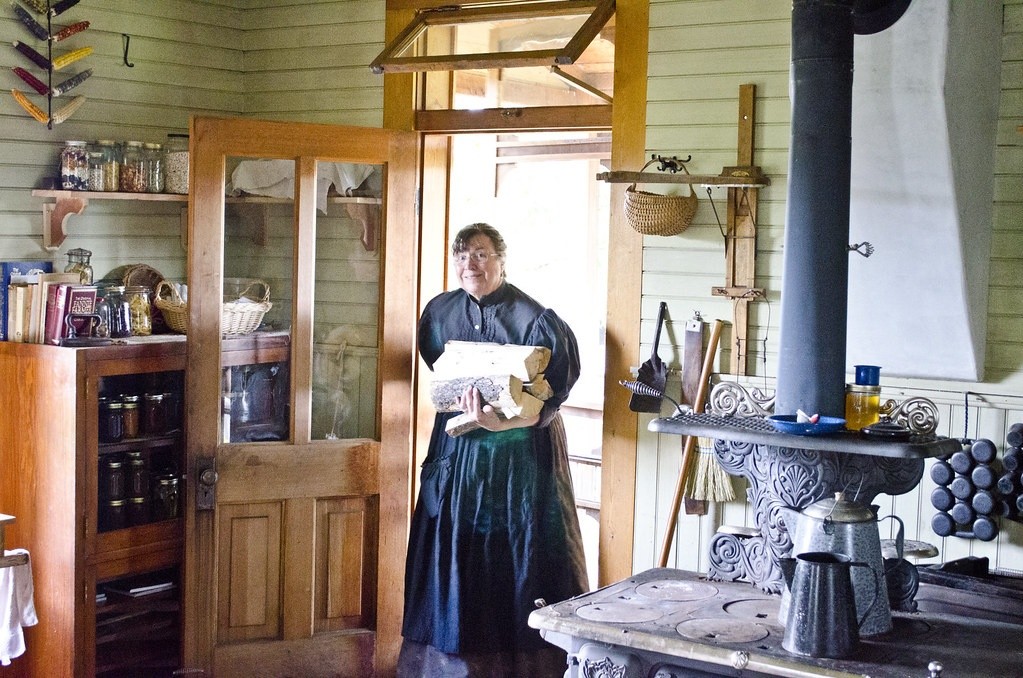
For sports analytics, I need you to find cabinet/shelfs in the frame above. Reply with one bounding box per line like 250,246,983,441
1,333,290,678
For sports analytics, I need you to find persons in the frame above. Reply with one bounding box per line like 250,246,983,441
401,224,588,653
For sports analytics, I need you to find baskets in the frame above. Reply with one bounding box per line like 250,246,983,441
624,156,698,236
154,278,271,335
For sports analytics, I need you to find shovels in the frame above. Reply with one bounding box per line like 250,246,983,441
627,300,670,414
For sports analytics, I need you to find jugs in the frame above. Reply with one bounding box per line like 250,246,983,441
778,551,878,660
778,470,904,635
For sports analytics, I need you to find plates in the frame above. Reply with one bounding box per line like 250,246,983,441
768,415,846,436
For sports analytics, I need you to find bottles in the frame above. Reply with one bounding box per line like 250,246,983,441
844,384,881,431
60,134,189,195
64,247,153,339
99,392,184,442
98,451,186,532
221,366,274,426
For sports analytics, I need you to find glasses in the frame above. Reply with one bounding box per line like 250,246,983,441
455,252,499,264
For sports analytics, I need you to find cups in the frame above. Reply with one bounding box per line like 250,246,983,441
853,364,882,385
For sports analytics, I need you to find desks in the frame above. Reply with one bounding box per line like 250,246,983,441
529,567,1023,678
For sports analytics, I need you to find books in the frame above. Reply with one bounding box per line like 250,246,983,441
0,261,97,346
